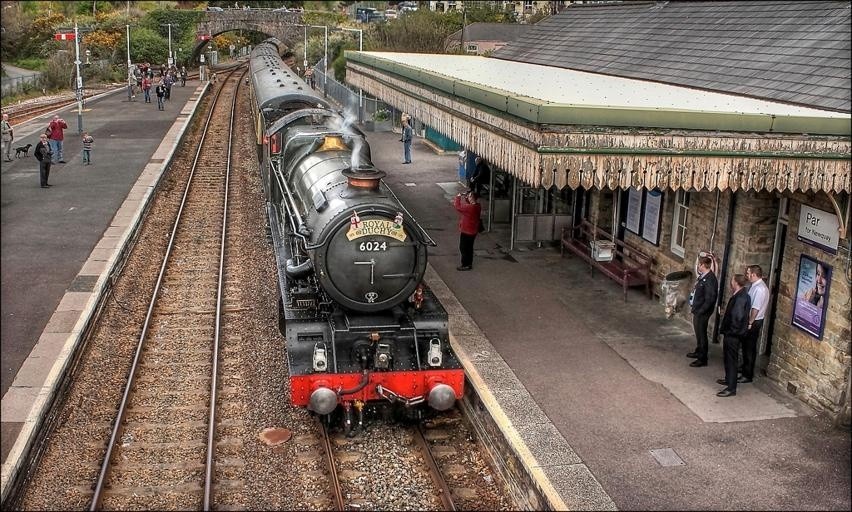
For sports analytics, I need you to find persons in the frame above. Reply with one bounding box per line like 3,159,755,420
799,263,829,309
466,156,490,195
130,62,188,111
453,190,481,272
736,265,769,383
1,113,14,162
34,134,54,188
304,68,316,90
46,115,68,165
82,132,94,166
398,112,411,142
716,274,751,397
686,257,719,368
401,120,413,164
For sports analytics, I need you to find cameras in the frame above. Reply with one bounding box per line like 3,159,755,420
461,192,467,196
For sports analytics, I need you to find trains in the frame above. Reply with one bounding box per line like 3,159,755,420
245,38,465,433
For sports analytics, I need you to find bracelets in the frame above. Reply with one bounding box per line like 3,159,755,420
747,324,753,326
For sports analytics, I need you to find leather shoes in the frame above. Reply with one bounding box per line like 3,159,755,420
717,366,752,397
457,265,471,271
687,353,707,367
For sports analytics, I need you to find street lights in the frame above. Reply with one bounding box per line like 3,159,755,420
59,22,94,133
302,23,329,99
280,22,308,83
330,27,364,126
159,24,180,70
197,27,221,68
116,24,141,102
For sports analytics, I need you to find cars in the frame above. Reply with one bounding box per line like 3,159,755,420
398,1,418,13
207,6,224,12
272,8,290,12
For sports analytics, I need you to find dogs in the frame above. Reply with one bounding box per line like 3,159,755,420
13,144,33,159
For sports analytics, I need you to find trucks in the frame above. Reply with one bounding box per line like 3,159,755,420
355,7,384,23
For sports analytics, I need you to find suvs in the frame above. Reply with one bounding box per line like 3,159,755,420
384,9,397,22
